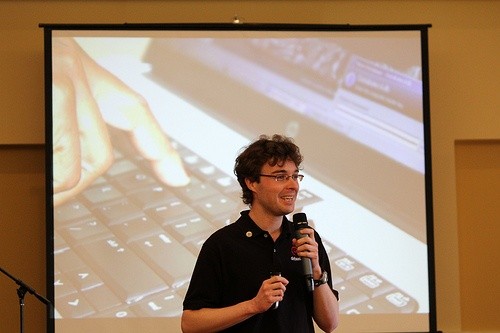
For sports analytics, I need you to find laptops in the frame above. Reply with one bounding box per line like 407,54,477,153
52,30,430,316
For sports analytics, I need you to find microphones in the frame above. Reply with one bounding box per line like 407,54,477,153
292,213,315,292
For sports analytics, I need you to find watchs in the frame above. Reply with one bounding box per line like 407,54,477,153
314,270,328,287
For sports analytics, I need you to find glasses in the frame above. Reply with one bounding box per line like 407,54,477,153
261,173,305,182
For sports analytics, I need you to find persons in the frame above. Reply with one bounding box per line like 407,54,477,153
53,38,190,207
180,135,339,333
